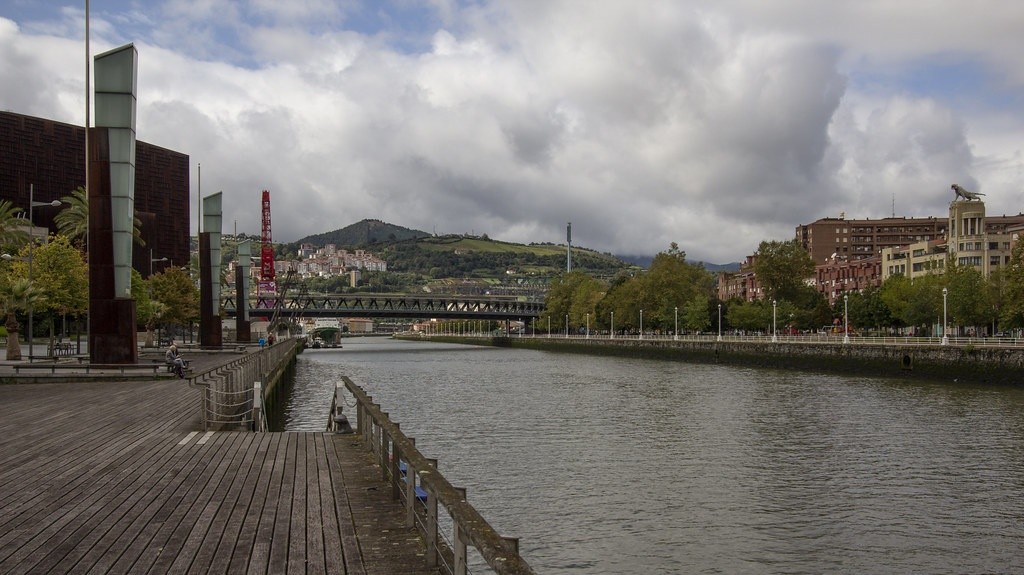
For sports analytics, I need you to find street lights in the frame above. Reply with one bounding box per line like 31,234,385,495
586,313,589,340
150,257,168,276
942,287,950,346
565,315,568,338
29,200,61,358
717,303,723,341
425,316,536,338
639,309,643,339
609,311,614,339
547,316,551,338
843,295,850,344
772,300,778,342
674,307,679,341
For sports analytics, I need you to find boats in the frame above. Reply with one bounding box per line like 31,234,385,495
312,342,321,349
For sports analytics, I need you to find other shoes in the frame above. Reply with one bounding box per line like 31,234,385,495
182,365,186,368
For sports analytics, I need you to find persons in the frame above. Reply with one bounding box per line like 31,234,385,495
259,336,267,350
166,345,188,378
268,332,274,347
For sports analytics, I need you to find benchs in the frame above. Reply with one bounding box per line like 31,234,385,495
137,338,268,352
0,355,193,373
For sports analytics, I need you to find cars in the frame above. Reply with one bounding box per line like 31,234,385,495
996,332,1011,338
